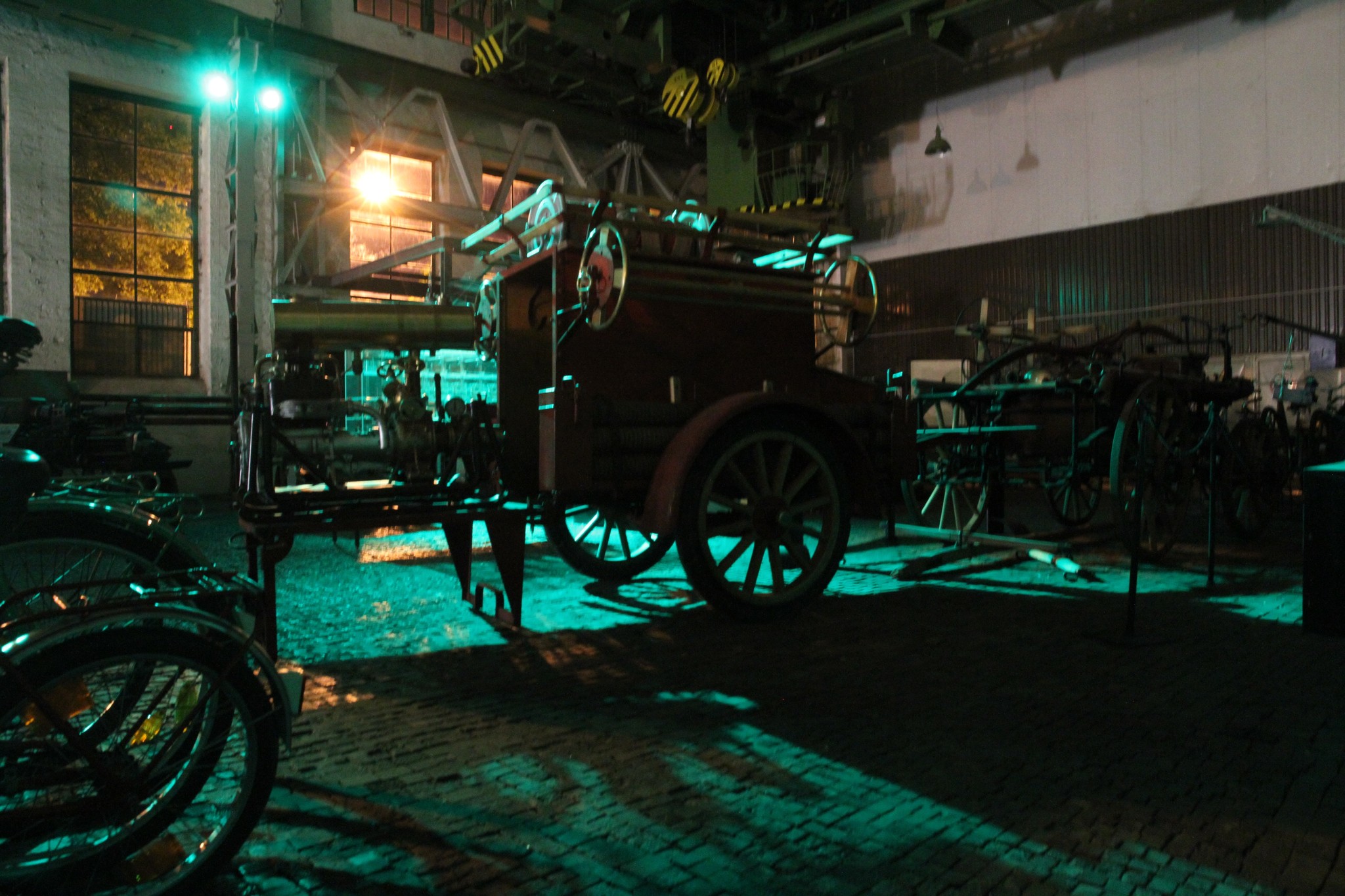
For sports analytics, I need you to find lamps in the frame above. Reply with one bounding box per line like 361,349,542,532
925,98,954,158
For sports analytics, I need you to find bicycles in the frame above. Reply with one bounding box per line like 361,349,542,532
903,310,1345,562
0,315,309,896
716,173,878,348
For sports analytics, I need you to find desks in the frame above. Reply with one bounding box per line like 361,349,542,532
1302,459,1345,633
